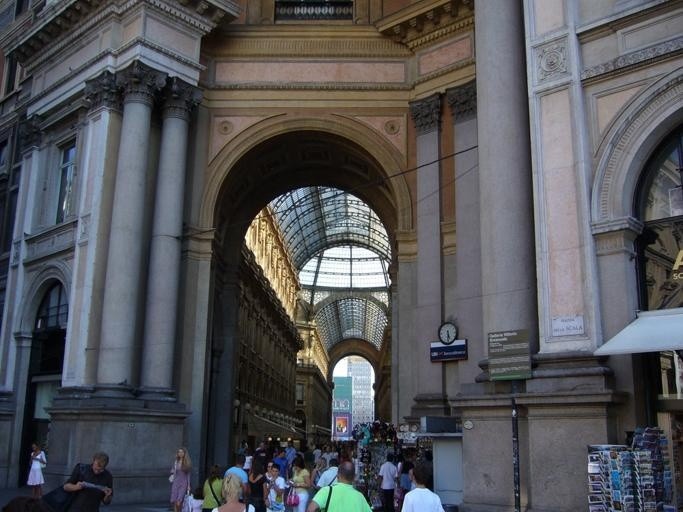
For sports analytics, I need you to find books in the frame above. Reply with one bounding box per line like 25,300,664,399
585,425,675,512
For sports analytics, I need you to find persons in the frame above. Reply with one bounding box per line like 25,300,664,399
169,444,193,511
56,451,114,511
25,439,46,499
184,436,449,512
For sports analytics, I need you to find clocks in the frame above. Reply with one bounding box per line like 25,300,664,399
437,322,457,345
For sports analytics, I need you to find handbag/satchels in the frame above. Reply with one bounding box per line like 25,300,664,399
41,463,47,468
167,473,176,483
181,490,195,512
286,492,302,507
269,501,284,511
